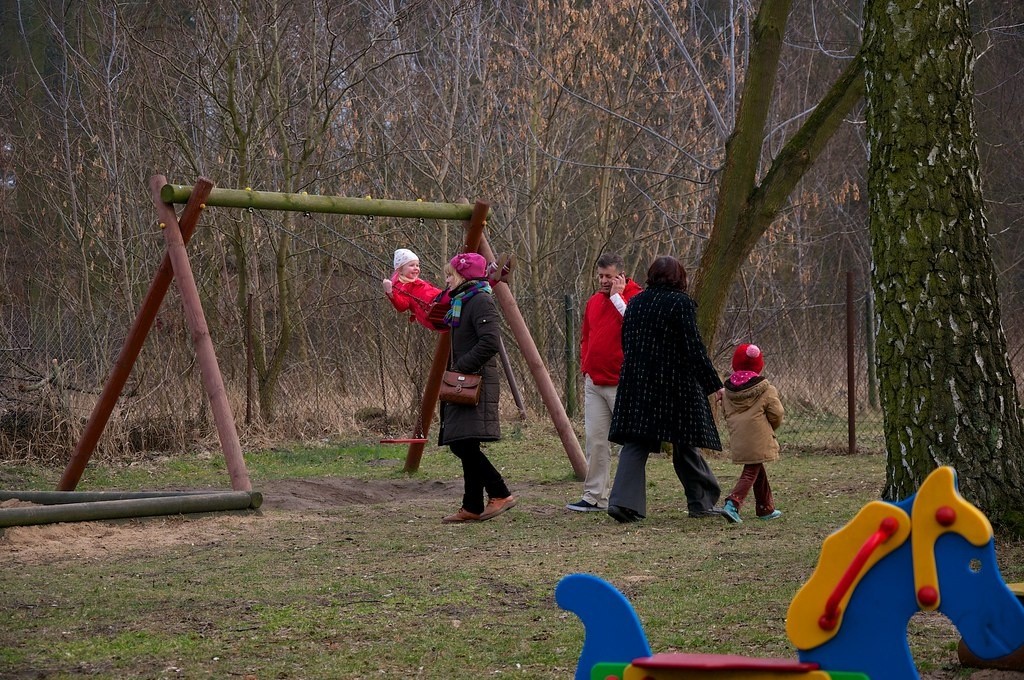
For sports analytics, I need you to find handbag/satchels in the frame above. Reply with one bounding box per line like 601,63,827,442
439,370,483,406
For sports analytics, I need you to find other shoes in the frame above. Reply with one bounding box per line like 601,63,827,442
688,506,721,517
608,506,638,525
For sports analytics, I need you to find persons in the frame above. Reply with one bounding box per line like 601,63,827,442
562,253,645,512
382,249,515,333
608,256,725,520
719,343,783,523
444,253,515,524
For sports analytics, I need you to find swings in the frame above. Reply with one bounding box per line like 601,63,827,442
248,205,453,323
368,214,430,444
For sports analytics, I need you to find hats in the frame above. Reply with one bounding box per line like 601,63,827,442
732,343,764,374
450,253,487,281
394,248,420,271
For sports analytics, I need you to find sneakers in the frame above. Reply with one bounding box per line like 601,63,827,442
442,507,479,524
489,253,506,282
722,500,743,523
501,253,516,282
760,510,782,521
479,495,518,520
565,500,607,511
